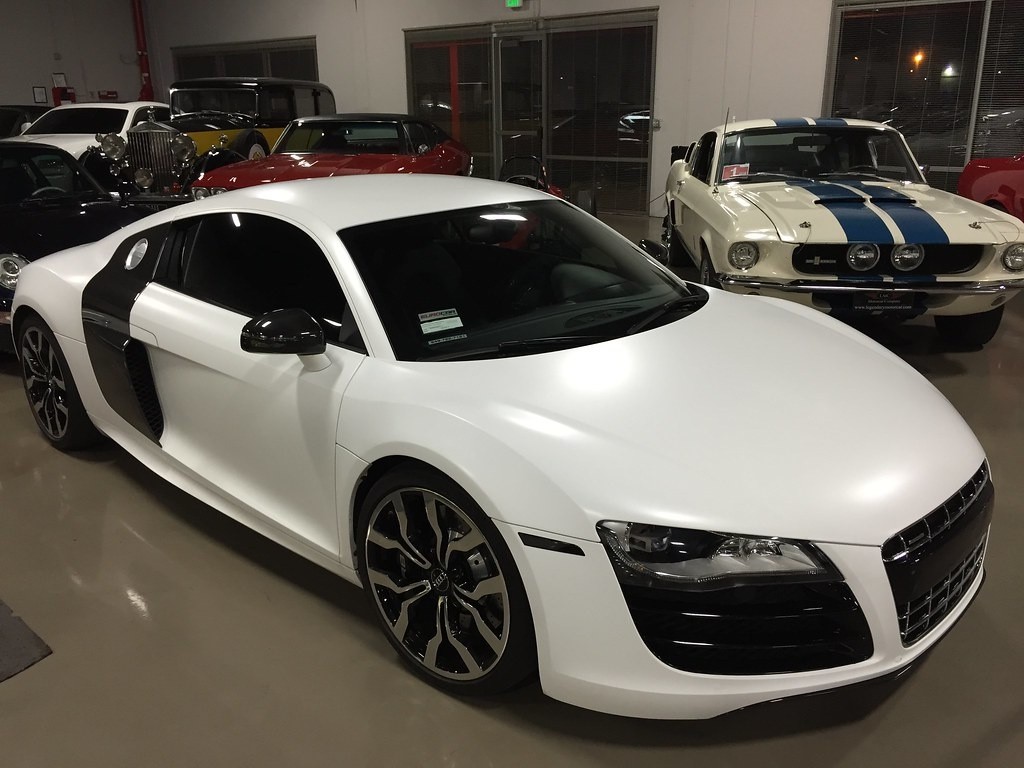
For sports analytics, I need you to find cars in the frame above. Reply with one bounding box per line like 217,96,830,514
617,109,651,154
664,116,1024,352
956,157,1024,224
0,77,338,377
190,112,476,202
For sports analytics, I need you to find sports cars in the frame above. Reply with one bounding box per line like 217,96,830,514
11,172,995,719
